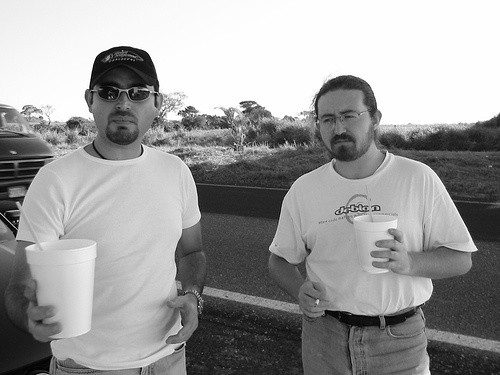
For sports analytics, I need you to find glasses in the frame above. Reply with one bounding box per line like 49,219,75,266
91,85,159,103
315,109,371,128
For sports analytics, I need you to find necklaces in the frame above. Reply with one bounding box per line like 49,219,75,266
92,140,143,160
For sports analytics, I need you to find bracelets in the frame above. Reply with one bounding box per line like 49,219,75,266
183,289,204,315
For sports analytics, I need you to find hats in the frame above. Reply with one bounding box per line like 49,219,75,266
92,46,159,87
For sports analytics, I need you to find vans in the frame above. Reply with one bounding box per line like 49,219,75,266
1,103,55,217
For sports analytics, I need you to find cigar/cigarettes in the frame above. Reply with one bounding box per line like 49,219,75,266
315,299,319,307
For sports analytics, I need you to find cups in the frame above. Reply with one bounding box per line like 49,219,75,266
353,214,398,274
24,238,97,340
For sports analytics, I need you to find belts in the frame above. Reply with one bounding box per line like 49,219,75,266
324,308,420,328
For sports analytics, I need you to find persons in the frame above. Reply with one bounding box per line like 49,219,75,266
267,76,479,375
5,45,208,375
